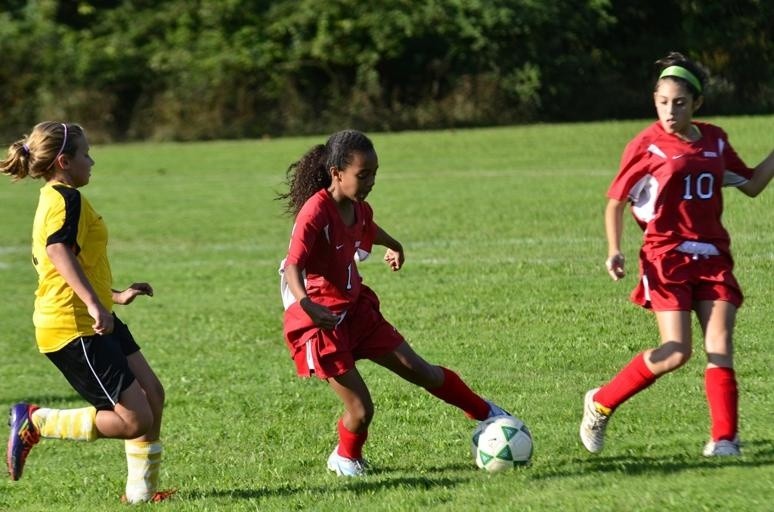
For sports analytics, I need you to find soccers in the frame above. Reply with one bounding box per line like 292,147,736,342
471,415,534,476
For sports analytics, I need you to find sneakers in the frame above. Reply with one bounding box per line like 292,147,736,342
119,492,174,506
7,402,40,482
580,388,611,454
703,434,743,458
326,443,367,478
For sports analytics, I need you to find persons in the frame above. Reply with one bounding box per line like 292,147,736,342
0,118,182,507
274,129,514,483
578,51,772,461
